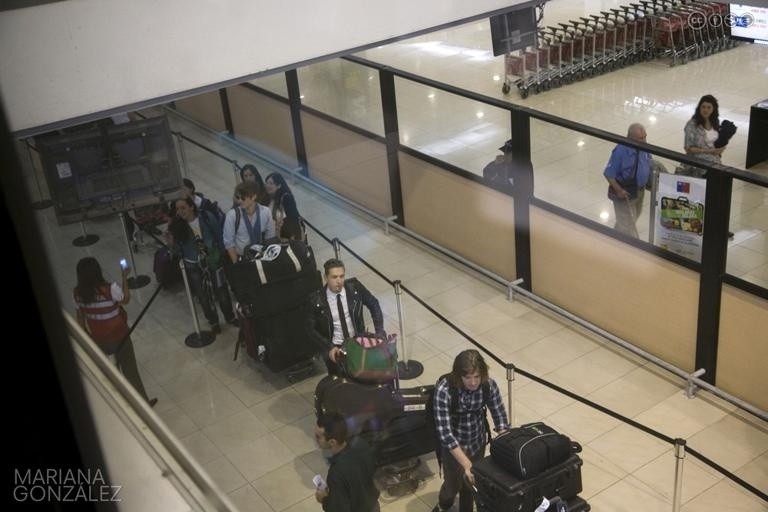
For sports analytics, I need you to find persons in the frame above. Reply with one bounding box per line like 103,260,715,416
433,350,510,512
305,259,384,377
482,140,533,198
124,161,309,331
680,94,734,238
72,256,159,408
315,412,380,512
604,124,653,242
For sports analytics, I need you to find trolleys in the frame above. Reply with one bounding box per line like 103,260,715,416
502,1,740,95
238,256,315,381
312,344,421,475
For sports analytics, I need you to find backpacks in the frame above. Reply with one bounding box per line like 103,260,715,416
425,373,491,451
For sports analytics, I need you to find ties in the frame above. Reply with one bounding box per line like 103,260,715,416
337,294,351,339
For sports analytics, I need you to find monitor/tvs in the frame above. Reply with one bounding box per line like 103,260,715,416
489,8,542,56
34,115,188,225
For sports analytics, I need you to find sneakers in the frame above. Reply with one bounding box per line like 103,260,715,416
211,323,221,334
228,317,241,327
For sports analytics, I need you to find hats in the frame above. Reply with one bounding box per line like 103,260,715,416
498,139,514,154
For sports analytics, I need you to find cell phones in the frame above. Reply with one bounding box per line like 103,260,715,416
119,257,129,270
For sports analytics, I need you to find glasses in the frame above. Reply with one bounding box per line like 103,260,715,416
234,194,247,201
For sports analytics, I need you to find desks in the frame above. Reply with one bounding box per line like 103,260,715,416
744,99,767,169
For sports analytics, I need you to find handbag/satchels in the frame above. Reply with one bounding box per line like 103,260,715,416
243,236,309,284
490,421,583,480
280,217,306,238
608,178,637,202
341,332,399,383
198,248,227,293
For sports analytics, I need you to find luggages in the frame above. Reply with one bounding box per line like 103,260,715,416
361,413,436,468
240,317,318,368
469,453,583,512
